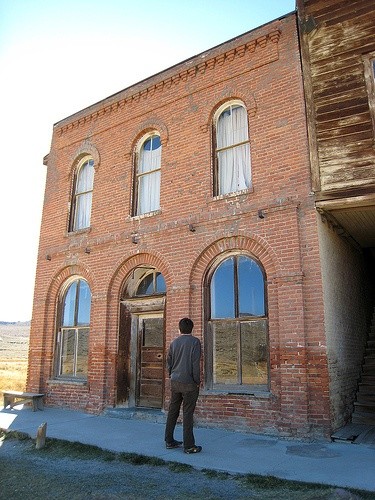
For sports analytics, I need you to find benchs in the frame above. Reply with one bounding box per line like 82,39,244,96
1,390,44,412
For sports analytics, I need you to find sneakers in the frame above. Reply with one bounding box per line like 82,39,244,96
184,446,202,453
166,441,183,449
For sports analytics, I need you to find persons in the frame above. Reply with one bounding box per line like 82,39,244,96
165,318,202,453
